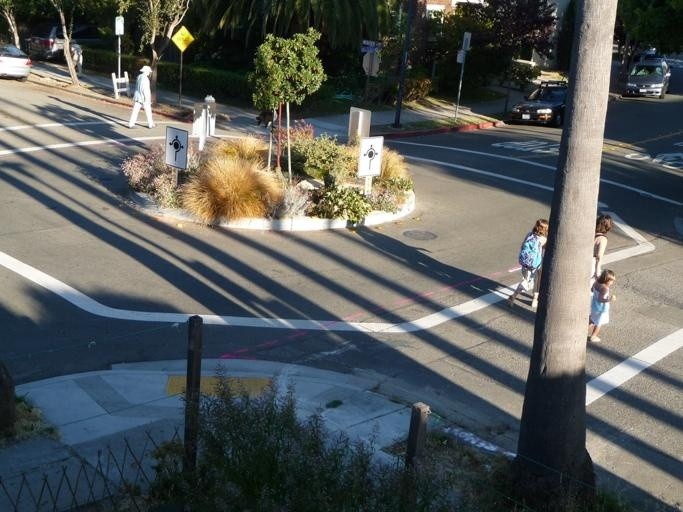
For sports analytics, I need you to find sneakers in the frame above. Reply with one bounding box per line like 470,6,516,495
590,336,600,341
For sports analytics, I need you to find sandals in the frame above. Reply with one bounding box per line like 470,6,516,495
129,126,138,129
149,123,157,129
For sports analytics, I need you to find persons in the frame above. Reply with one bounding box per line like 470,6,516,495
590,213,612,294
66,41,86,79
129,65,155,129
507,219,549,307
589,270,616,342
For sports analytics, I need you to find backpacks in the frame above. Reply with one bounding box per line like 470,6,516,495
518,234,544,272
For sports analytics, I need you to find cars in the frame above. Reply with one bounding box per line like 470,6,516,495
510,80,569,127
0,43,32,80
625,57,672,100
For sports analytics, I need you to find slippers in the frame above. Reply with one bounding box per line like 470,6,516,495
507,296,514,308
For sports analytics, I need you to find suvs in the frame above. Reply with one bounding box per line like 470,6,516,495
31,24,115,61
